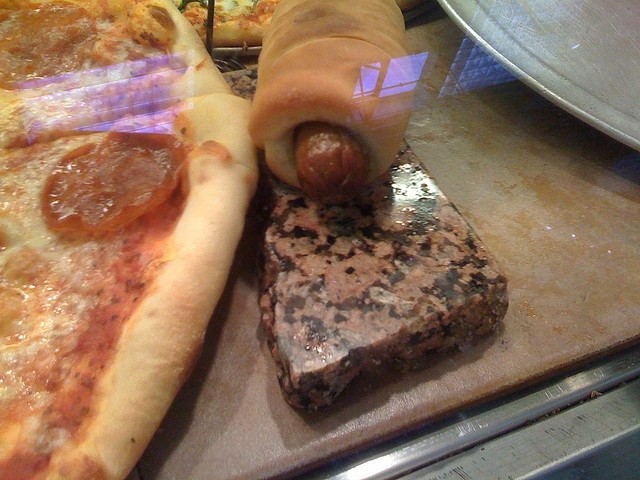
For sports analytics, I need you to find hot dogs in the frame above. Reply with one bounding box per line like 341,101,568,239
247,2,419,196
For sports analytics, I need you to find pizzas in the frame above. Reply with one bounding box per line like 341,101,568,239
170,2,281,47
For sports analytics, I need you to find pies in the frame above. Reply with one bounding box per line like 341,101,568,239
0,2,260,478
